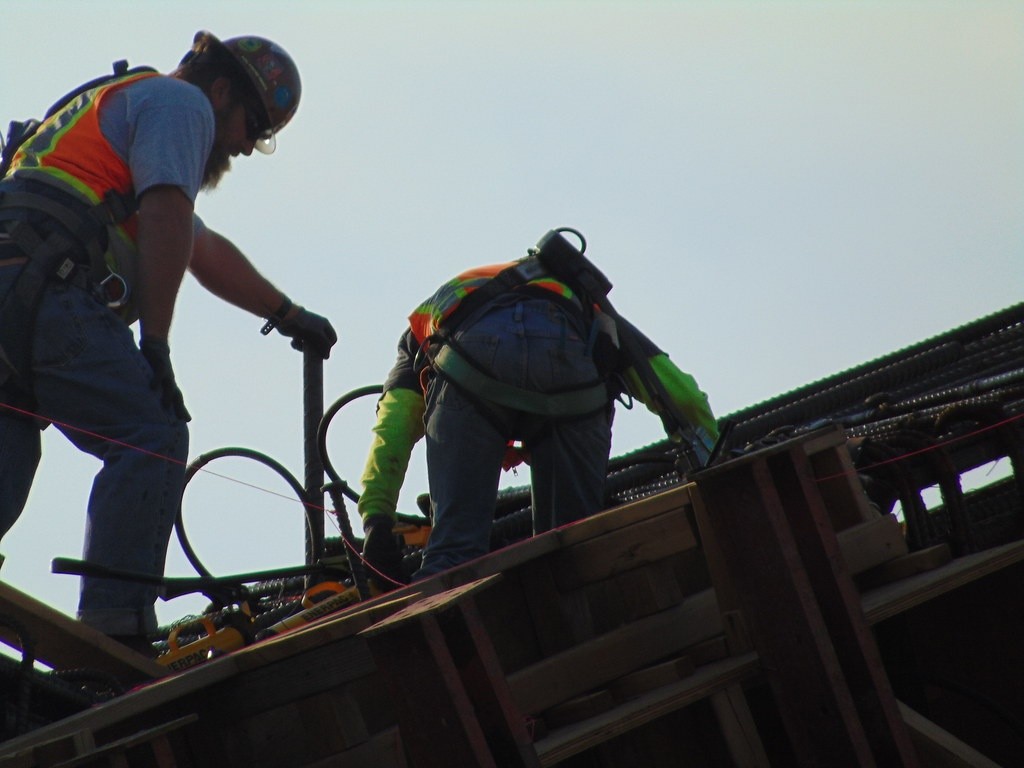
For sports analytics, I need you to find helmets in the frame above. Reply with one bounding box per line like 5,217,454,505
193,30,301,154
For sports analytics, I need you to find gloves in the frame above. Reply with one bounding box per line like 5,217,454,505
275,306,337,360
139,335,193,422
360,514,408,574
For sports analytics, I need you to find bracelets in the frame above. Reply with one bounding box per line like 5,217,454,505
260,295,293,336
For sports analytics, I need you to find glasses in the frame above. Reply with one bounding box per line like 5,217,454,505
244,96,263,141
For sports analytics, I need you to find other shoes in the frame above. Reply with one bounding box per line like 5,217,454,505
114,635,161,660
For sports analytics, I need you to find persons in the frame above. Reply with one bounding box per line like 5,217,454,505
357,260,732,602
1,26,339,698
